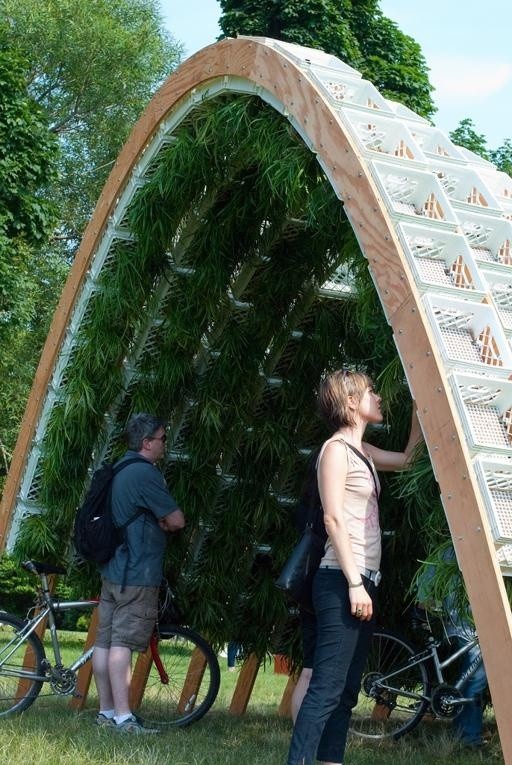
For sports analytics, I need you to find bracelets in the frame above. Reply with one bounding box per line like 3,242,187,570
347,581,364,589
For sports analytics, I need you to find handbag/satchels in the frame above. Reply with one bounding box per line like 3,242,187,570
275,528,323,614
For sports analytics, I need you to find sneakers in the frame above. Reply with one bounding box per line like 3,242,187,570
95,712,160,735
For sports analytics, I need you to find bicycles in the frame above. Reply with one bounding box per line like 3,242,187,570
347,606,483,742
0,555,220,731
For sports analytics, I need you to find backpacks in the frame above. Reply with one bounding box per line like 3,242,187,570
74,457,153,565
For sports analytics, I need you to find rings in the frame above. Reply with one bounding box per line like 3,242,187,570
357,608,362,616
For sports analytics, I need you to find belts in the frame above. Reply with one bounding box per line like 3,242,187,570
319,559,382,586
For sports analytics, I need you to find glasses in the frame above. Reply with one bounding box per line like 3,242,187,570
152,435,167,443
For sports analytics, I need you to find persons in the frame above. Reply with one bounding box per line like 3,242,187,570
286,366,425,765
87,412,187,733
